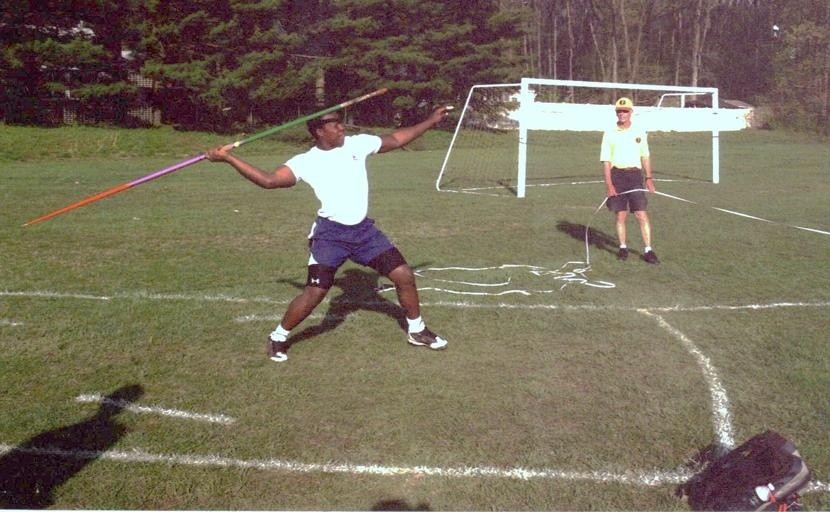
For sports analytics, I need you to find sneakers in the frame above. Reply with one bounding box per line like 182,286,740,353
407,327,448,350
644,251,657,264
267,331,288,362
619,248,629,259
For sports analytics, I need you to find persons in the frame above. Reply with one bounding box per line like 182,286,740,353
201,104,458,362
601,97,661,265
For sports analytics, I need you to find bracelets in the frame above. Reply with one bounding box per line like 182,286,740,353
643,175,654,182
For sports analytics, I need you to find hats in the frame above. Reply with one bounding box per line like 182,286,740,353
615,97,633,111
